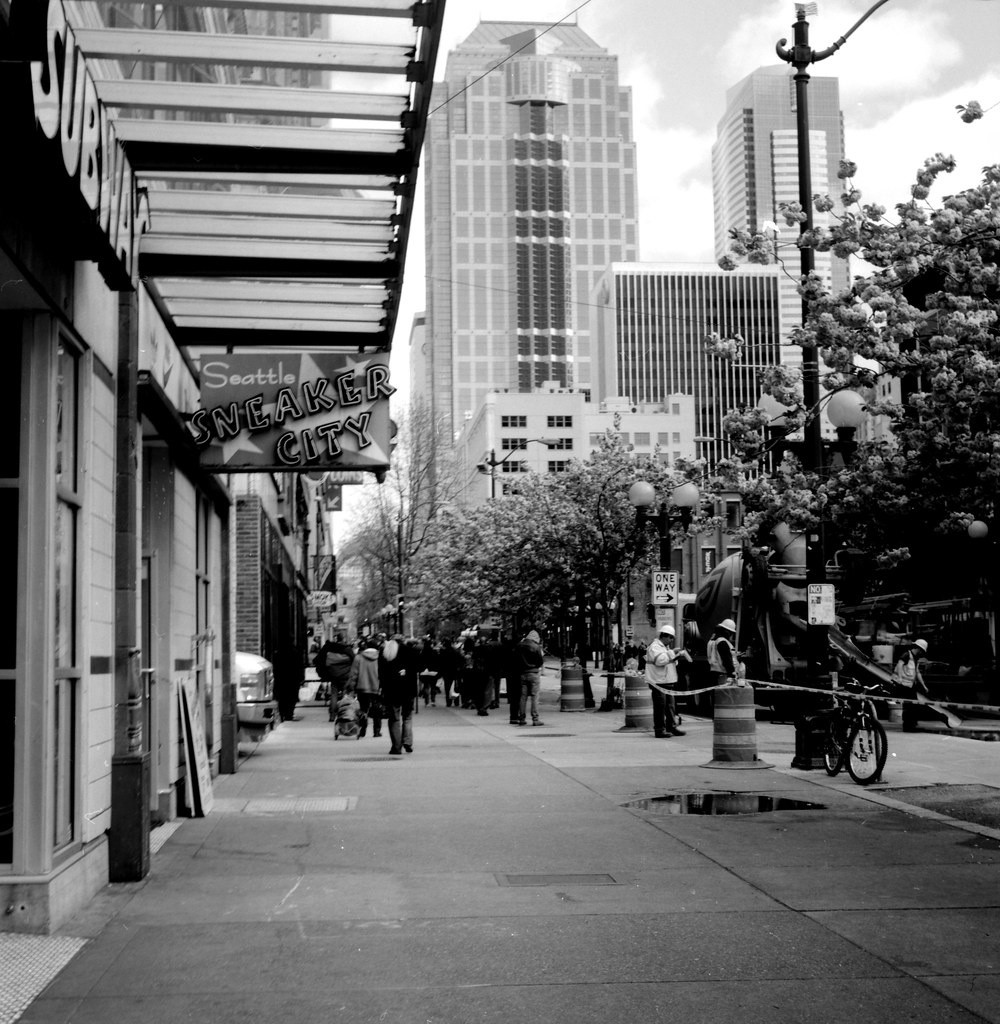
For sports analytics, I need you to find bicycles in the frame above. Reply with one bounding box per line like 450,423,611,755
821,682,889,786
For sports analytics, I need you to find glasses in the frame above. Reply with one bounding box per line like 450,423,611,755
668,635,674,639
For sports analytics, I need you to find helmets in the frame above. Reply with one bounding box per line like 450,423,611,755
657,624,677,637
911,638,928,653
719,619,736,632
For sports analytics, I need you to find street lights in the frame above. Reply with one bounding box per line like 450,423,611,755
488,435,561,587
757,385,868,768
397,500,450,633
628,480,701,570
595,601,603,668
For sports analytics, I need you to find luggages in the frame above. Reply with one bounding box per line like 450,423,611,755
335,692,360,739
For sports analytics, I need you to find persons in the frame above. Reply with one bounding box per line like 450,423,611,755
603,641,647,688
643,625,693,738
706,619,743,723
310,630,546,754
272,632,304,721
891,639,929,732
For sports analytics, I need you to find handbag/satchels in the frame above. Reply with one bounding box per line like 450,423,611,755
447,678,463,699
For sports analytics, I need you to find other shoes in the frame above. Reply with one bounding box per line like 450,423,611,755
519,721,527,725
404,744,412,752
510,720,520,724
389,750,402,754
666,727,685,736
478,711,488,716
903,726,920,733
533,720,544,726
359,721,367,736
374,733,381,737
654,728,672,738
